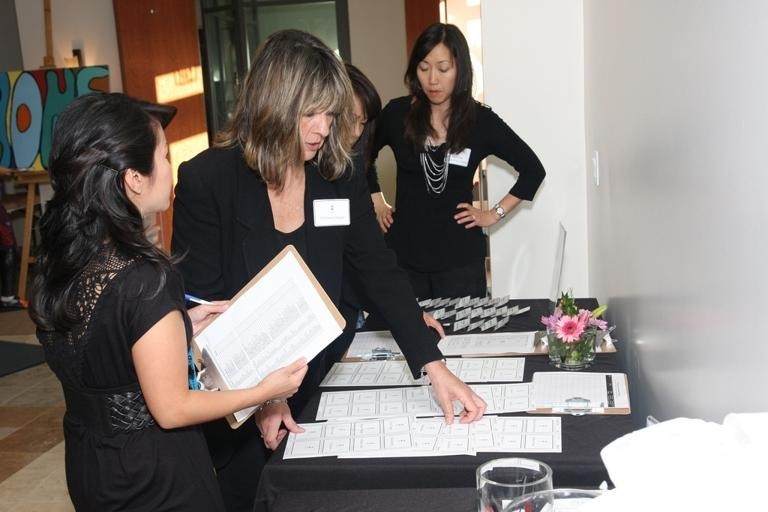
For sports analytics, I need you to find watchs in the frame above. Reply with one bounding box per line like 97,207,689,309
495,202,505,220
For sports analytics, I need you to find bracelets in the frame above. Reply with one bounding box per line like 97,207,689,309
257,397,287,412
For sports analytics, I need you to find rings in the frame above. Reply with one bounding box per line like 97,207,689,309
261,433,265,439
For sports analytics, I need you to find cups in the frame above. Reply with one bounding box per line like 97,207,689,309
476,456,553,512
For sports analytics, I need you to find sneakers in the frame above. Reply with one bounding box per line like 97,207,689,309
0,296,28,312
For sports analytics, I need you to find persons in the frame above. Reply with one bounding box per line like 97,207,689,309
171,28,488,512
312,61,446,341
354,22,546,297
27,92,309,512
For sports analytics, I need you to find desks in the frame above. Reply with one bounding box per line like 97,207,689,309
252,298,637,512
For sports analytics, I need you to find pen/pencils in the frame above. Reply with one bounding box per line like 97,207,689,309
184,291,213,307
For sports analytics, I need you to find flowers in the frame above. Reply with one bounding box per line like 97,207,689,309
541,289,608,355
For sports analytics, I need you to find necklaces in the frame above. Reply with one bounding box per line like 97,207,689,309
419,136,452,194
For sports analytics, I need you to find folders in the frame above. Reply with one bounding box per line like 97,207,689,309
544,324,619,355
341,329,407,364
525,370,632,419
459,330,547,358
188,244,346,431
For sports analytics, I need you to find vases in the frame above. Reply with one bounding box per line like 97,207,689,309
546,331,597,369
